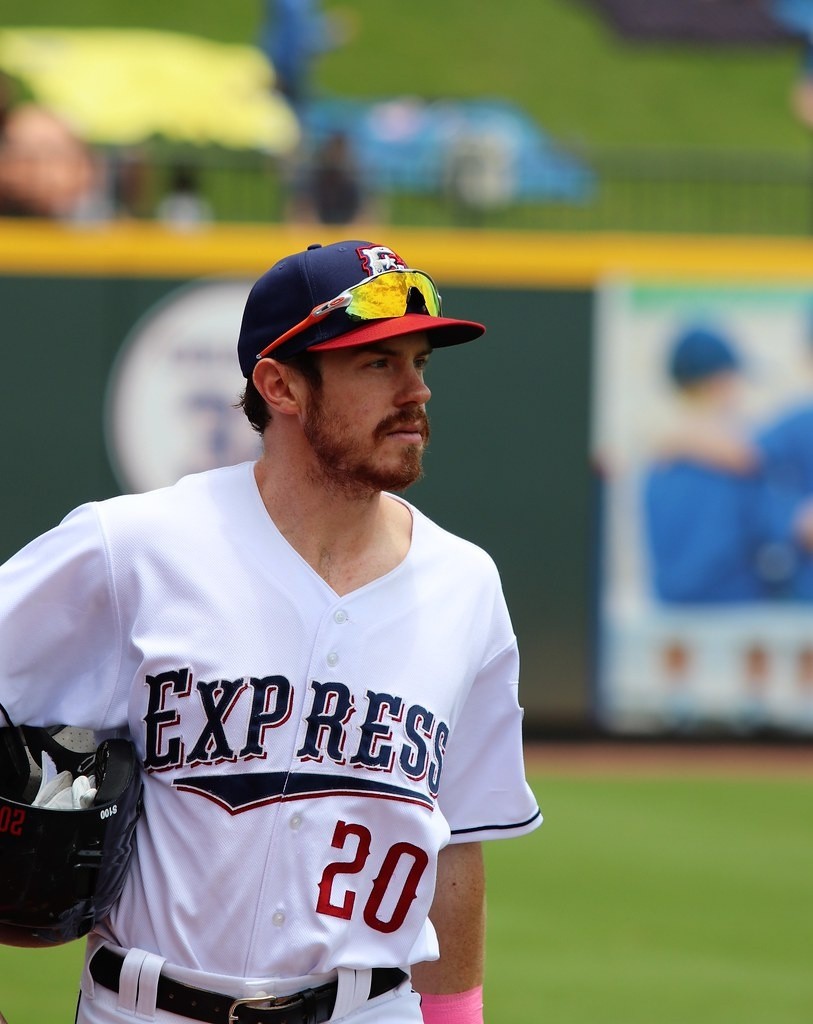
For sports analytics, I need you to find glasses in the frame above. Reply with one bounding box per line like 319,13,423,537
256,269,441,360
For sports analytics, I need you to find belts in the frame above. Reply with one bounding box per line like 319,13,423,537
85,947,408,1024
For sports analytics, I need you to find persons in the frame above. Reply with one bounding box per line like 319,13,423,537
633,294,813,612
0,95,499,230
0,238,546,1024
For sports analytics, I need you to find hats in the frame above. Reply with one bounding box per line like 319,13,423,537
236,239,486,379
671,332,734,384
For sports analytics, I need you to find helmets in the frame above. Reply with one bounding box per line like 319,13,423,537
0,708,147,951
